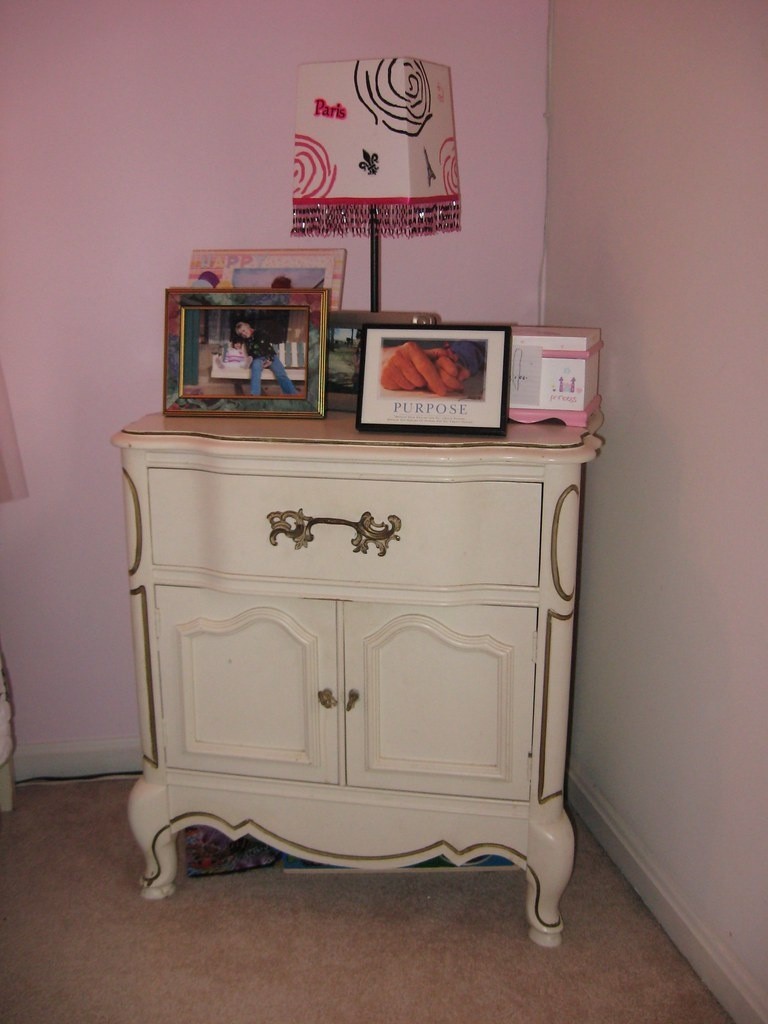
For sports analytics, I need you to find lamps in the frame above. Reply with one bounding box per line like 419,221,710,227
289,57,462,312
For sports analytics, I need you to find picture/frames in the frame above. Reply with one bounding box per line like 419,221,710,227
186,246,346,309
164,288,329,419
355,322,513,437
324,310,438,413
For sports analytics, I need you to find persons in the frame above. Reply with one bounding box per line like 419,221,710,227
270,276,294,289
234,322,300,395
380,340,485,397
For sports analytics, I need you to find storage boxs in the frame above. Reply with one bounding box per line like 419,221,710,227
508,326,602,428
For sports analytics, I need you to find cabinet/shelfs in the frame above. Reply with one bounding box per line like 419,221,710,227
112,409,604,946
